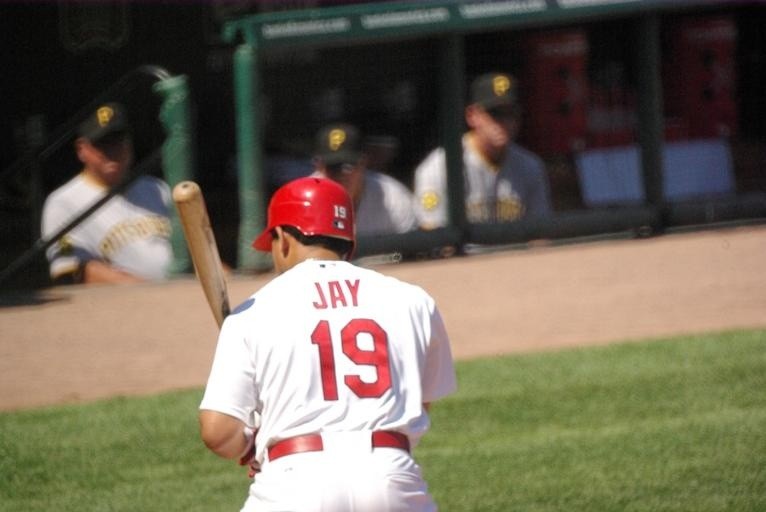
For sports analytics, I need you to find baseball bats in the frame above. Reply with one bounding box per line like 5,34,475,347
172,180,231,330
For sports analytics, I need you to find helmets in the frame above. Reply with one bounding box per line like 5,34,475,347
251,180,355,252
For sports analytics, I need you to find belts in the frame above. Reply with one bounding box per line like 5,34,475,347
265,431,412,460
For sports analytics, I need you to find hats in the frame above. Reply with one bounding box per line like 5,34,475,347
80,100,131,142
474,69,527,113
306,124,368,168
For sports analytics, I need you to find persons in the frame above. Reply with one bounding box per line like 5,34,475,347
38,99,195,290
260,122,418,258
197,175,459,512
413,69,556,257
